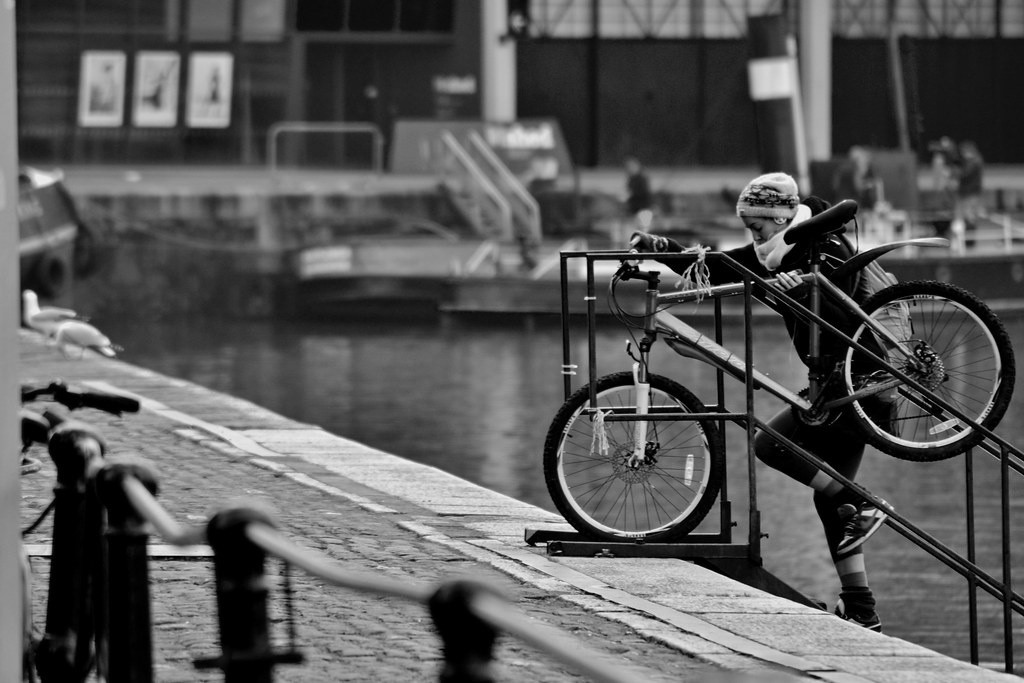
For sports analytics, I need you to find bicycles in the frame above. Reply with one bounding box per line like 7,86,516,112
541,197,1018,544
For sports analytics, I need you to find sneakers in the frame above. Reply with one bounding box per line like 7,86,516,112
834,485,893,554
833,585,881,633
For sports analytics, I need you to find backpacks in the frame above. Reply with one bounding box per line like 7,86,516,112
842,234,913,351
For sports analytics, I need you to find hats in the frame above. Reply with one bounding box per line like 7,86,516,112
737,172,799,220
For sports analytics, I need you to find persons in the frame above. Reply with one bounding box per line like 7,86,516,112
950,141,984,247
621,156,652,217
629,173,899,630
